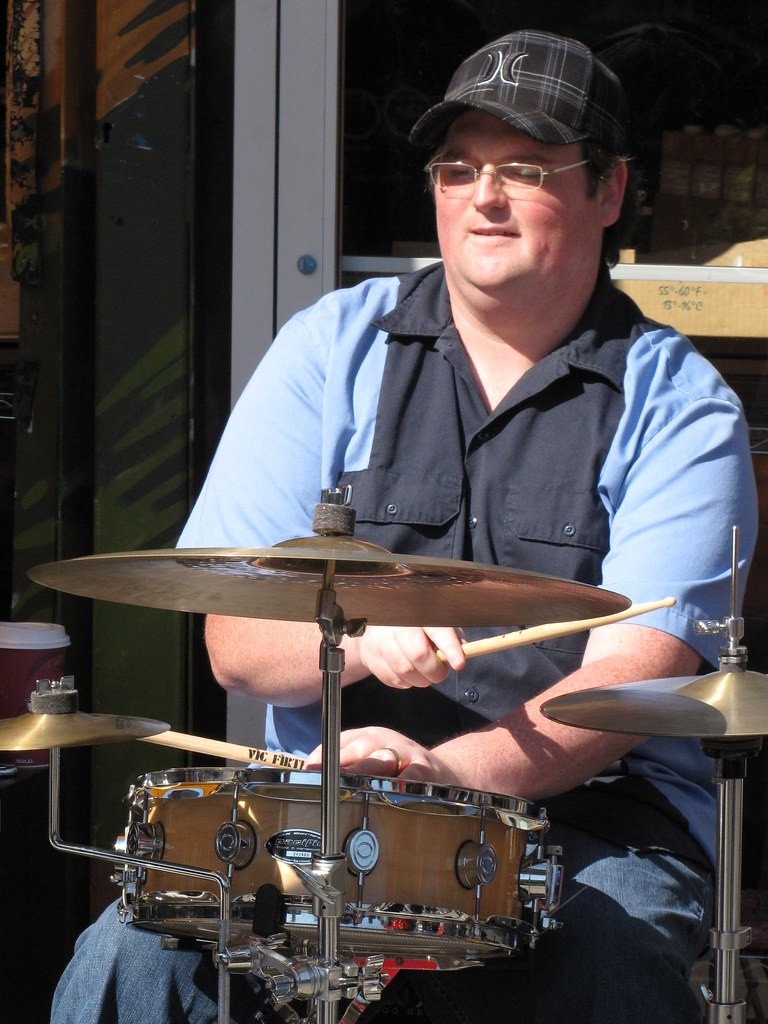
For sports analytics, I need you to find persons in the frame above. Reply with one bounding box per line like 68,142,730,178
50,30,759,1024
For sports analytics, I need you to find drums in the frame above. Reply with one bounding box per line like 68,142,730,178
119,762,569,969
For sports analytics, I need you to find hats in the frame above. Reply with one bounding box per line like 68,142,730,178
403,26,639,170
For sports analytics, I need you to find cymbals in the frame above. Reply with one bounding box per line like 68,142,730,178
20,525,640,631
536,666,768,743
0,708,173,753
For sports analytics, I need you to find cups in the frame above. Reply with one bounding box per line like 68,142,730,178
0,622,72,767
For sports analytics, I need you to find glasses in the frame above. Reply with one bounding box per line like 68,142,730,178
421,160,589,197
